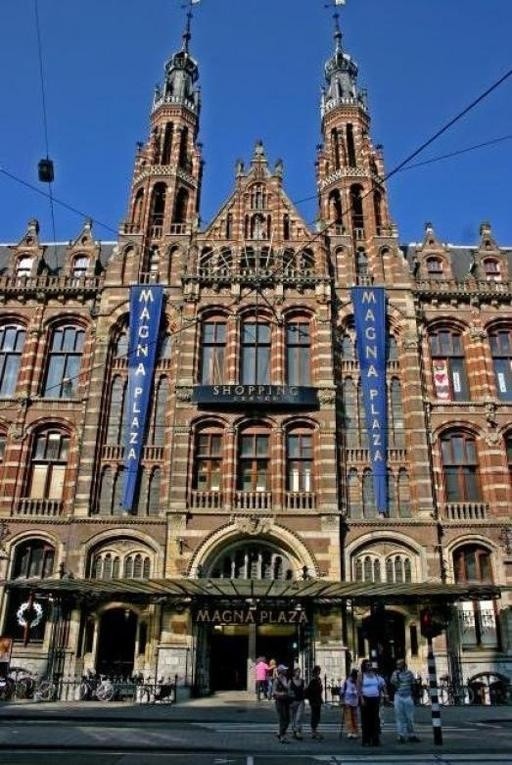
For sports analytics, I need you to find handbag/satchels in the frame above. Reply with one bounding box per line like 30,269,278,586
272,688,288,700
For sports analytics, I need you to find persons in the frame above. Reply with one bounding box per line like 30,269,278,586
1,636,13,689
255,655,422,748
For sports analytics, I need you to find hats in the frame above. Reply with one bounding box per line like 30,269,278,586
277,664,289,672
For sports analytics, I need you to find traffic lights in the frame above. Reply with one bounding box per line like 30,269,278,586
420,605,442,639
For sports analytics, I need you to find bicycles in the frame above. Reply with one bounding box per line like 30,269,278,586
11,666,58,702
383,675,430,706
133,676,175,707
75,674,116,702
439,674,474,707
